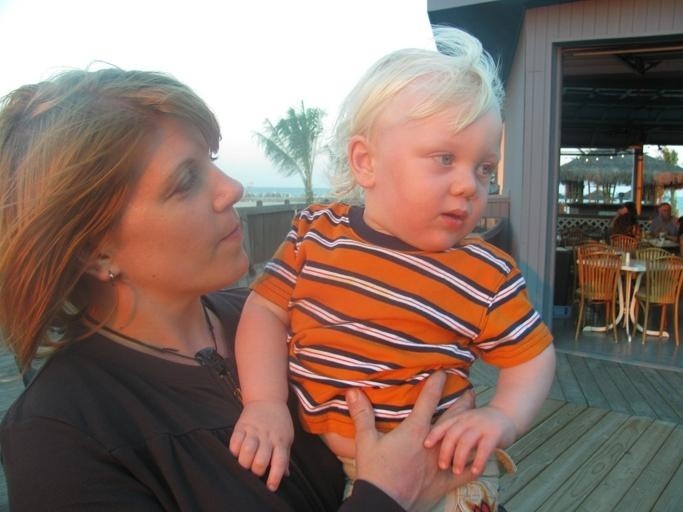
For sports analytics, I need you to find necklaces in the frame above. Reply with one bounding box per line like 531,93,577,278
81,299,244,410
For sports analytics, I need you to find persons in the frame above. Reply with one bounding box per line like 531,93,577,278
0,63,482,512
648,203,681,241
609,202,641,240
228,25,558,512
678,216,683,257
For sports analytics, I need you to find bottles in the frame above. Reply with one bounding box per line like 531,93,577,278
626,252,631,266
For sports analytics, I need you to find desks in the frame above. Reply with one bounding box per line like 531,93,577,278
556,247,569,266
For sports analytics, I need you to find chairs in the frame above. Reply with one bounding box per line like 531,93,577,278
572,233,683,347
